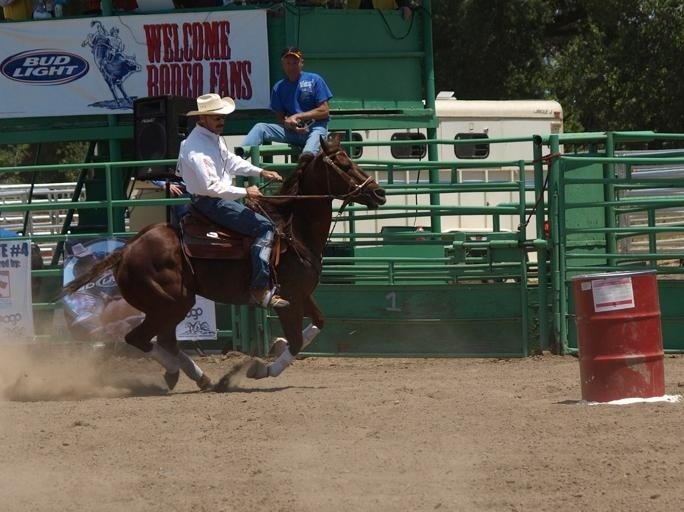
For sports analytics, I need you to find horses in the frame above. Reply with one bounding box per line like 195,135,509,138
46,131,389,391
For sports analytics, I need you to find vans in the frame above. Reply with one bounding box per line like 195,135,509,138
125,88,564,250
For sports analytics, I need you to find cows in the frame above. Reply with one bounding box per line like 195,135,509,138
80,19,143,108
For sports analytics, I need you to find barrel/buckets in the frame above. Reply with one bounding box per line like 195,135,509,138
572,271,666,402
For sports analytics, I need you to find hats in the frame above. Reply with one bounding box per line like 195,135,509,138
280,47,303,61
72,244,93,257
186,94,236,116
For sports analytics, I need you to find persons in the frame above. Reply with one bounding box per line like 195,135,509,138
139,165,184,198
234,47,334,163
179,93,293,310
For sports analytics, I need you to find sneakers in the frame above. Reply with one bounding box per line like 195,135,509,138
250,285,290,309
298,151,315,164
